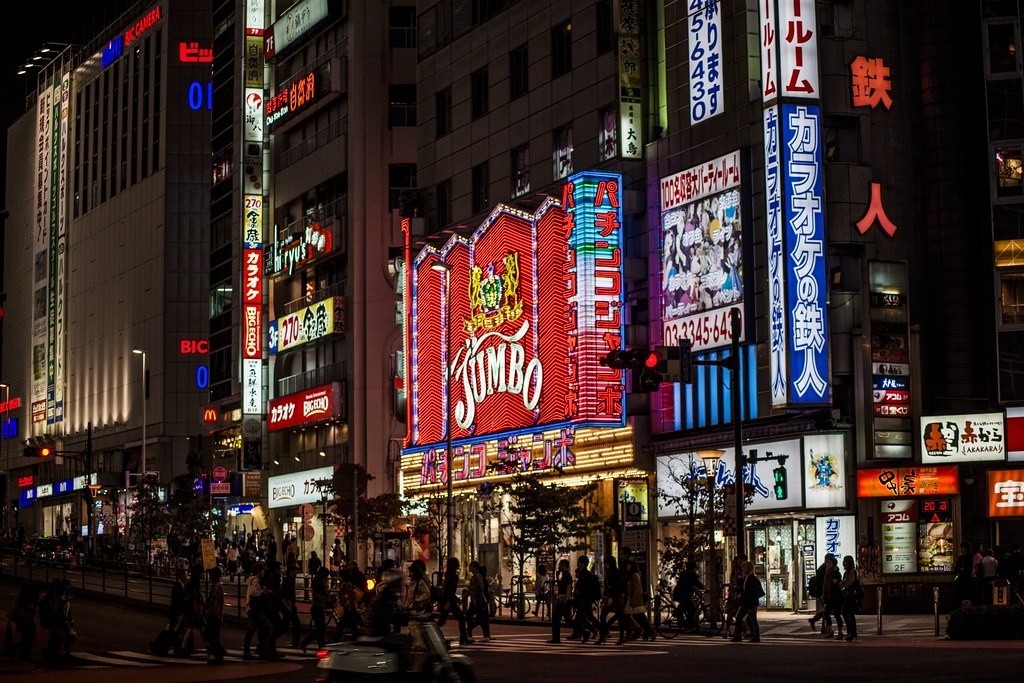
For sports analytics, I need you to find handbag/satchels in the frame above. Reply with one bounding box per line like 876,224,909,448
846,568,865,602
427,585,441,603
249,578,268,609
412,576,431,611
67,623,79,645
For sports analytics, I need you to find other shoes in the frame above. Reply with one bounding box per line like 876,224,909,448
747,637,760,643
532,611,538,616
459,639,475,645
731,638,741,642
820,628,826,634
824,633,833,639
835,633,843,640
547,625,656,646
175,639,307,665
809,619,816,631
845,634,857,641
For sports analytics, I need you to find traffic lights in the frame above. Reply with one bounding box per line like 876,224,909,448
23,446,51,458
11,503,19,512
607,350,639,369
636,350,663,393
773,467,786,500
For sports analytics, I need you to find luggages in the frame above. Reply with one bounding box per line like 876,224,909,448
149,614,185,657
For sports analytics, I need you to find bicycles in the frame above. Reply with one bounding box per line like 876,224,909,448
654,587,725,639
486,585,531,617
555,581,599,621
309,578,367,645
113,548,189,577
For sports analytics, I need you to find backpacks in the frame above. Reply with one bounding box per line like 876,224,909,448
807,567,823,598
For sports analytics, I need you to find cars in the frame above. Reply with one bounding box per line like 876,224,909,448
47,541,87,571
20,537,41,568
31,538,62,567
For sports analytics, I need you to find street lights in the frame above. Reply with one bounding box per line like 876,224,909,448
430,261,453,560
132,349,147,550
0,383,11,545
696,448,726,631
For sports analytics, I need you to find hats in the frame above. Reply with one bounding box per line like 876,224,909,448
207,567,222,579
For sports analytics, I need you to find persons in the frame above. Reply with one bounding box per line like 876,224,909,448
807,551,865,643
2,526,501,664
532,551,764,647
952,540,1024,610
